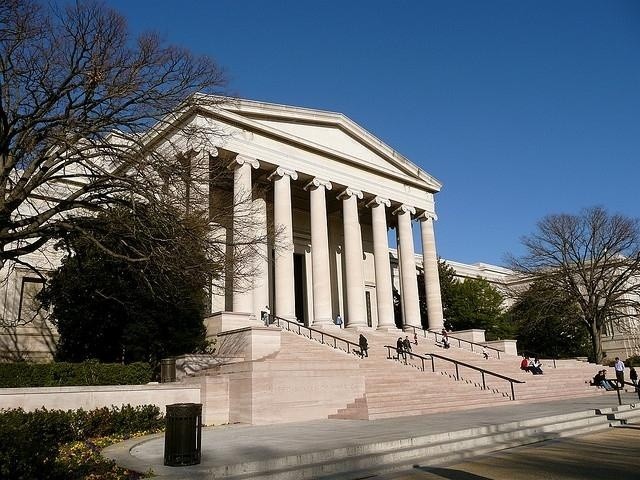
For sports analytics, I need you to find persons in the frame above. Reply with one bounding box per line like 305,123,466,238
442,329,447,336
397,337,405,359
521,356,539,375
482,346,488,359
530,357,543,374
414,334,418,344
335,315,341,328
263,306,269,327
403,336,413,359
443,332,450,348
359,334,368,357
594,357,638,393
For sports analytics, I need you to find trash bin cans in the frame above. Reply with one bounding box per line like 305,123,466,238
161,358,176,383
164,403,203,467
